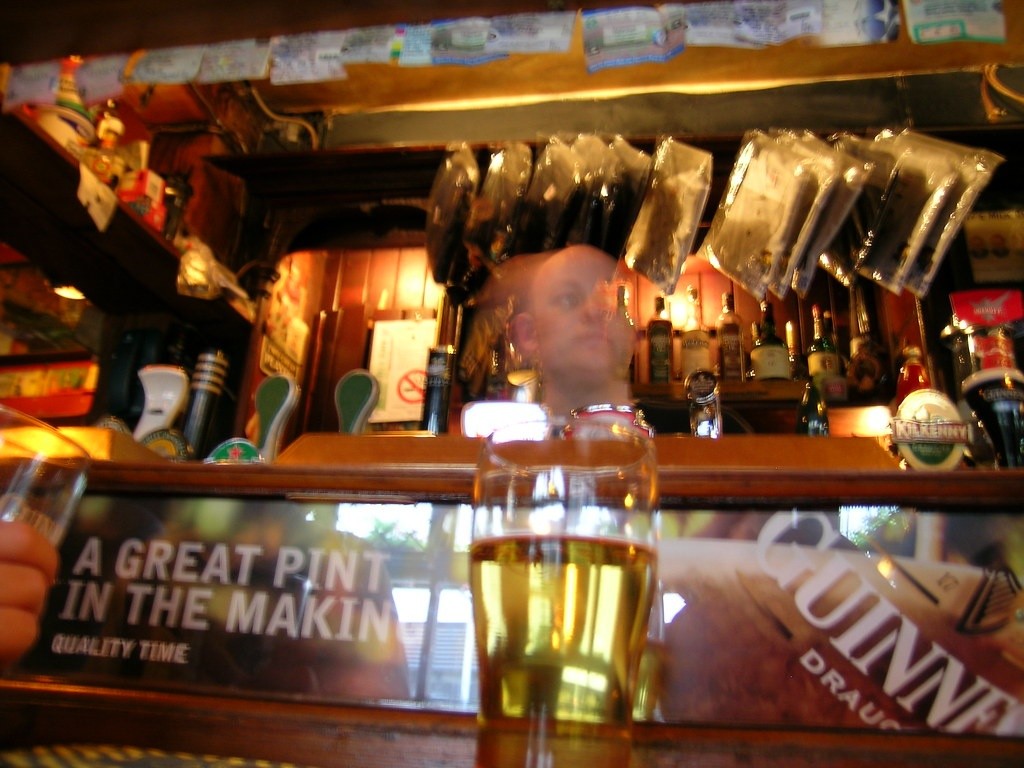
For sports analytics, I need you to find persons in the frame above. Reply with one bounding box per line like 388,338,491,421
511,245,753,434
0,517,59,666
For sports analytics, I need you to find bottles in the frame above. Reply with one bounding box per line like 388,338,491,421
607,284,888,381
795,378,828,436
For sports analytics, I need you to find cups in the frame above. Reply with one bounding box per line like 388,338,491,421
961,367,1024,469
462,431,667,727
0,410,90,548
